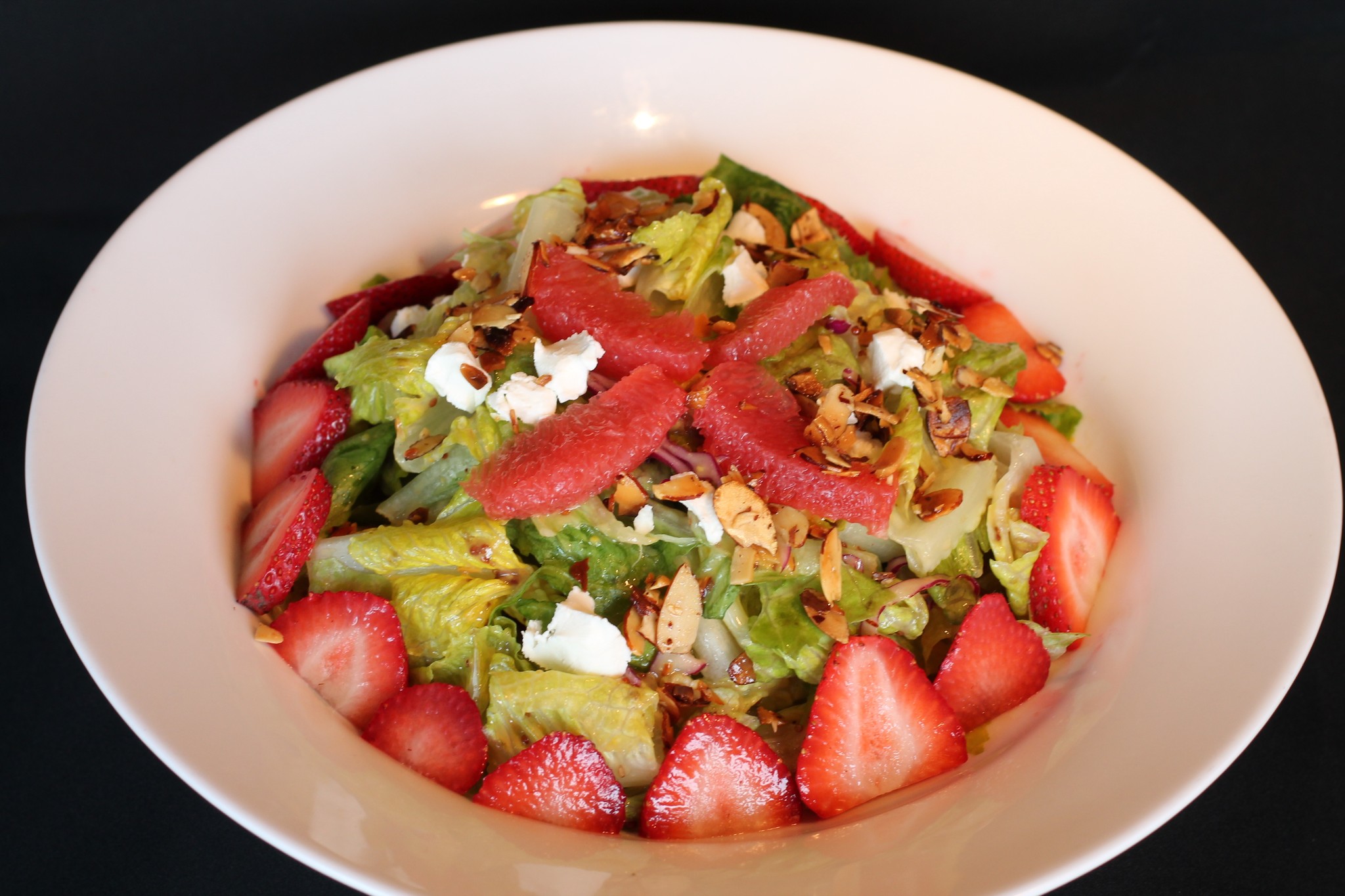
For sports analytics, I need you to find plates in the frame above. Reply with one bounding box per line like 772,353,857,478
19,17,1343,896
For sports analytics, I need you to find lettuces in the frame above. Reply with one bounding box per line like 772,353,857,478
301,154,1081,817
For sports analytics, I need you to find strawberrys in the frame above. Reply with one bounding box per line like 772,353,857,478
1020,461,1120,651
874,228,988,311
995,408,1114,500
278,297,371,385
794,632,969,816
252,379,352,503
265,587,409,732
640,712,801,841
958,299,1067,406
324,266,459,327
236,465,333,612
932,593,1052,734
792,193,871,257
581,175,703,204
474,732,626,837
362,681,489,793
